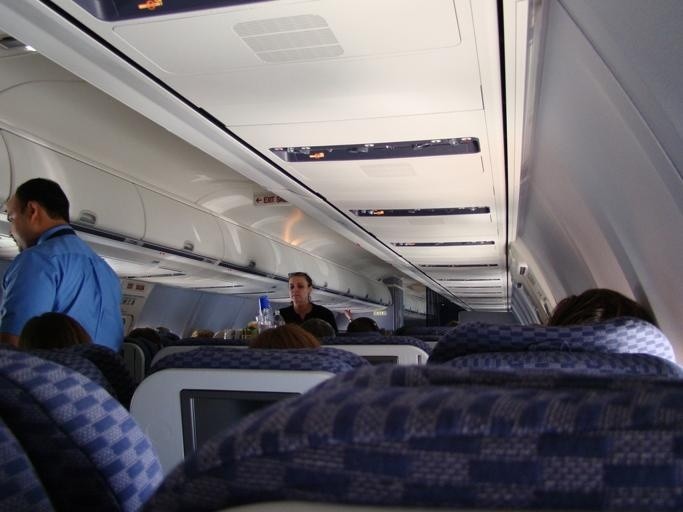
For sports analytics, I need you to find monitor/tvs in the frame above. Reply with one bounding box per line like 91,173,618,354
361,355,400,373
177,388,301,460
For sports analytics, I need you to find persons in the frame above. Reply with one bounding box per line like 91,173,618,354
549,288,657,325
245,317,459,349
0,179,128,352
20,311,214,365
276,272,338,335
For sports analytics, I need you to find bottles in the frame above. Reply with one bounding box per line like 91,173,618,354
258,308,274,334
273,310,285,329
